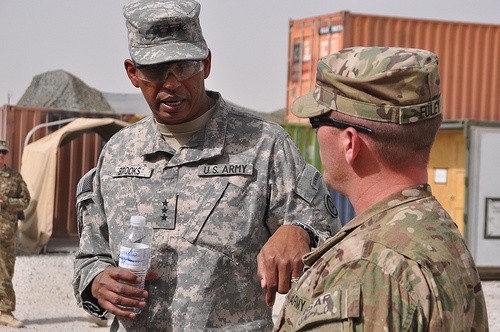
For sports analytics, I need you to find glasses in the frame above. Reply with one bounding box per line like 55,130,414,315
0,150,7,154
136,61,205,84
309,116,373,135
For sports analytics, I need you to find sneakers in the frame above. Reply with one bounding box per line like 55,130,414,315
0,311,24,327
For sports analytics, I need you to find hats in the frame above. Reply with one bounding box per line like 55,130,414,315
124,0,209,65
291,46,443,125
0,140,9,152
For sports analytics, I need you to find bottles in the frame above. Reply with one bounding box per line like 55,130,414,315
116,215,150,314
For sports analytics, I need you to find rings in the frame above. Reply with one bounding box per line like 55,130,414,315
291,276,300,283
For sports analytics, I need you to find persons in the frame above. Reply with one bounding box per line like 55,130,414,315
0,139,31,328
274,48,491,332
73,0,343,332
